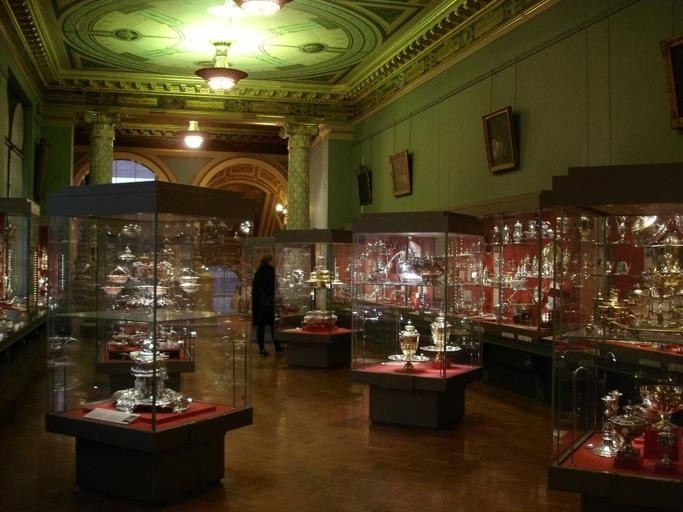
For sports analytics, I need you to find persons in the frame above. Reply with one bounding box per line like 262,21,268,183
250,253,288,357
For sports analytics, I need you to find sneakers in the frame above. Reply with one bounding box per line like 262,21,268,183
260,346,286,356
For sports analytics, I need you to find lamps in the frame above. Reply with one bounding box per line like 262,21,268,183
233,0,294,15
175,121,207,148
194,48,247,92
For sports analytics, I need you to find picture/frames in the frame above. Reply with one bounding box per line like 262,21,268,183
36,137,53,199
357,149,411,204
659,34,683,129
481,105,519,173
